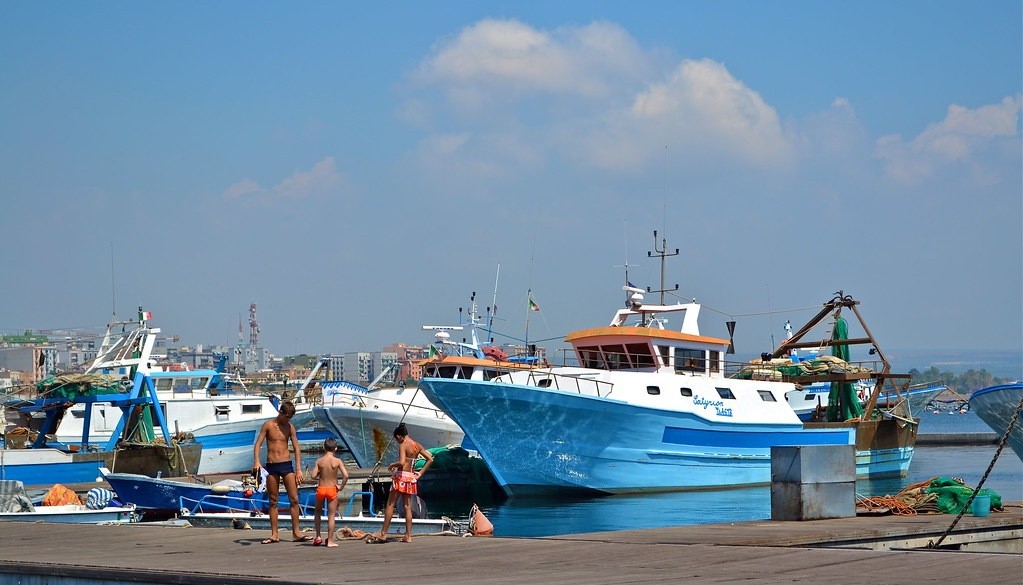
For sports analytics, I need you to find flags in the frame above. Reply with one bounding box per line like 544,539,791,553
529,299,539,312
138,312,152,320
429,346,436,358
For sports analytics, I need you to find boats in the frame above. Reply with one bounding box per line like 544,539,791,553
311,262,552,469
179,490,471,535
968,382,1023,463
288,419,349,453
0,305,323,523
784,320,948,423
416,229,921,497
97,464,341,522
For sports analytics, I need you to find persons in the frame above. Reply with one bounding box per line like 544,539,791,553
254,401,314,544
312,439,348,548
372,423,433,542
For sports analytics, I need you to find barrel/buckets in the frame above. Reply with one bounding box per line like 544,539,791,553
973,495,991,516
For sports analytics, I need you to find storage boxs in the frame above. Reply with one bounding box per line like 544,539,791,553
770,444,857,520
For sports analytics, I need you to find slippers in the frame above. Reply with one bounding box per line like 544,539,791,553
325,538,328,544
293,535,313,541
261,537,279,544
312,539,322,546
